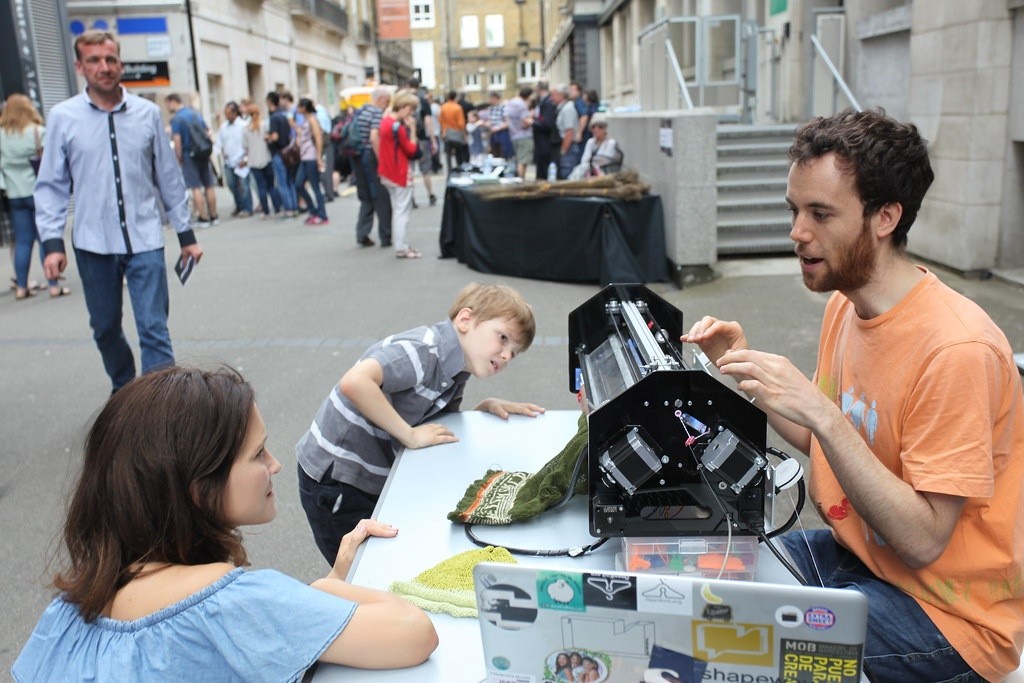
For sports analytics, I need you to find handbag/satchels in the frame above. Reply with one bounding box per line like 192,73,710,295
280,142,300,169
446,129,464,146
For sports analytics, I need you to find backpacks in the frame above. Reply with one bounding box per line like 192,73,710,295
174,115,212,161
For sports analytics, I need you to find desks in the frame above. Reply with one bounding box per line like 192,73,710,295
309,411,870,683
438,167,672,289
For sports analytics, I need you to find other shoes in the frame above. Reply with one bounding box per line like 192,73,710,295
358,237,374,247
194,207,309,227
429,196,437,204
381,241,392,248
396,246,422,258
304,215,328,227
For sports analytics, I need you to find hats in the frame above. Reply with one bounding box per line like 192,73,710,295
588,112,608,128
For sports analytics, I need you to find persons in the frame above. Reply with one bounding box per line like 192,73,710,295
327,79,441,259
565,112,621,182
32,30,203,398
678,108,1024,683
11,366,439,682
437,79,602,180
0,94,70,298
218,90,340,225
165,92,220,226
295,280,545,568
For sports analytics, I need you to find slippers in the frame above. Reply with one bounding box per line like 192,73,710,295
16,288,38,300
50,286,71,297
